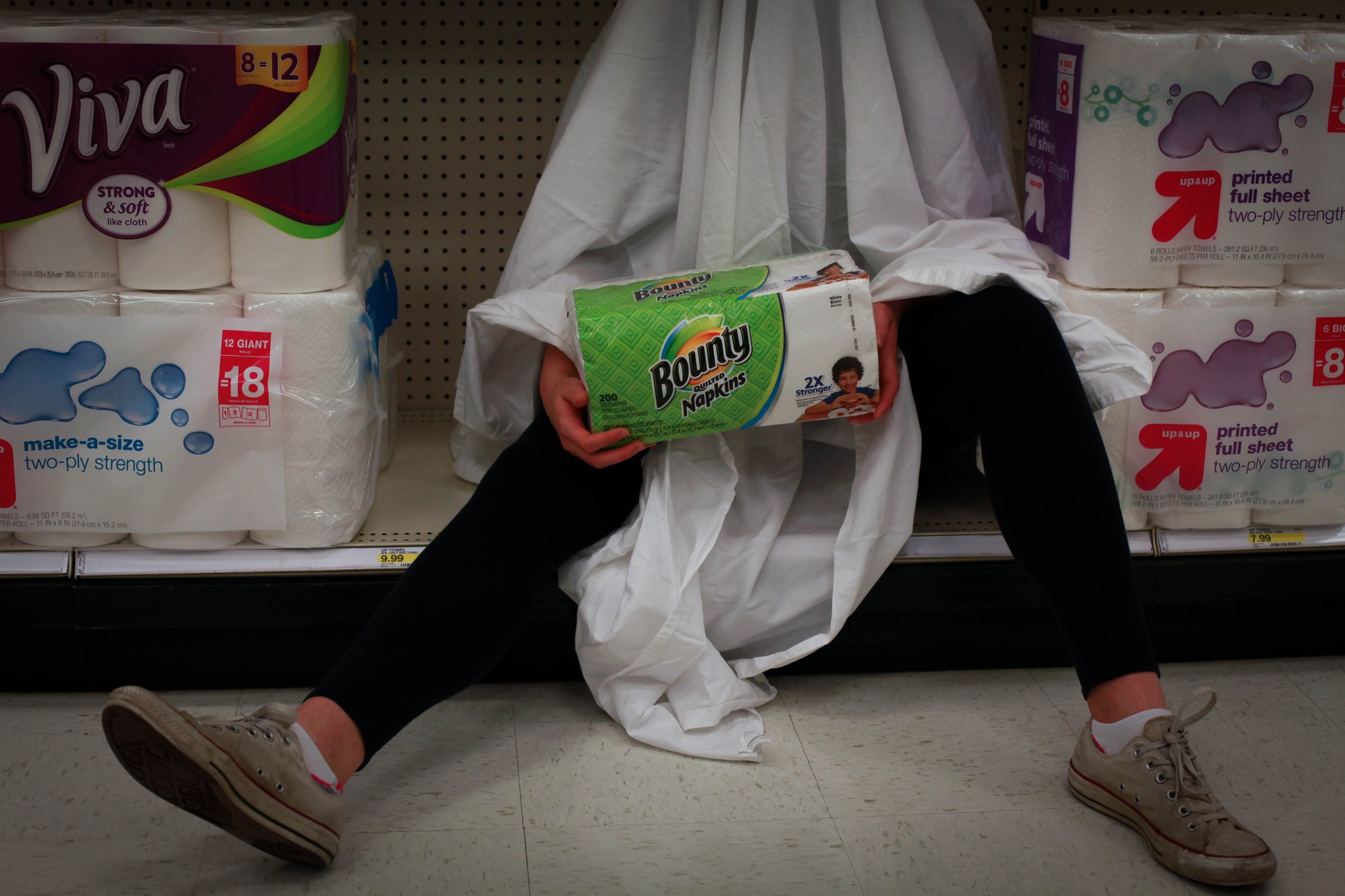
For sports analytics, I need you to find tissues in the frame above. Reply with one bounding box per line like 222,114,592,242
562,247,887,455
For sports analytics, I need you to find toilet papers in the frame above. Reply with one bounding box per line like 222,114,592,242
977,1,1345,538
0,0,401,554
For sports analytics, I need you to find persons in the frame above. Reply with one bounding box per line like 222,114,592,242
98,1,1282,893
793,261,867,287
804,356,880,415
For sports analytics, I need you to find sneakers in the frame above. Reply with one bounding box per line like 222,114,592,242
105,687,344,867
1069,709,1278,883
